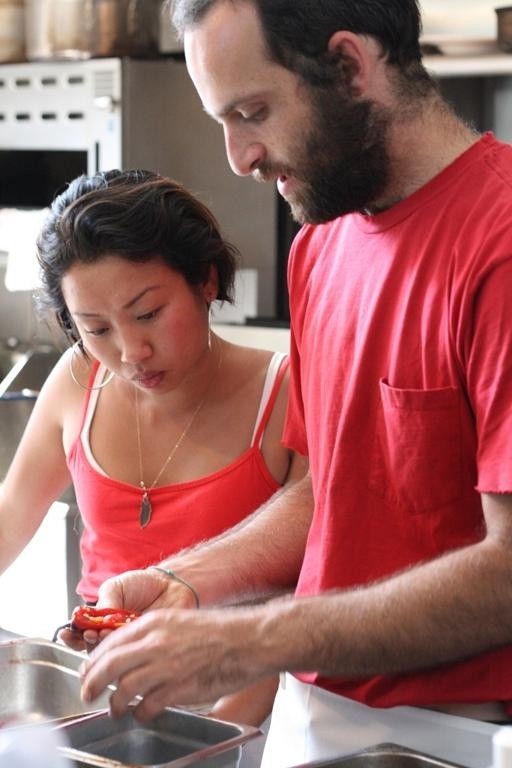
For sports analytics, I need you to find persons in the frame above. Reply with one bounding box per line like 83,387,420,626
76,0,512,768
0,167,294,767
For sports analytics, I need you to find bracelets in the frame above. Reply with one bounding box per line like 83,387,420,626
144,565,201,610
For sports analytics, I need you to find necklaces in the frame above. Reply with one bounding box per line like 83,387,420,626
133,341,225,531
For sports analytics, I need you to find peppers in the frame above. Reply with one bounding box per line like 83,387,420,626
51,605,142,643
